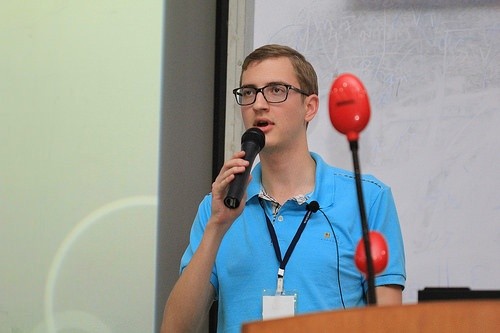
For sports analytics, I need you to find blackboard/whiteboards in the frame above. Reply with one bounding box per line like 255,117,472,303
223,0,499,309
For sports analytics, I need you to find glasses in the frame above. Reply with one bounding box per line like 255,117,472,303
233,84,310,105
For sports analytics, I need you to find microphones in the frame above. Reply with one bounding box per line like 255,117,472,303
223,127,265,209
309,201,320,213
355,232,388,305
328,73,377,305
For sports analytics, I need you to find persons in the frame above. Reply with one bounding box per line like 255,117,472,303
160,43,406,332
